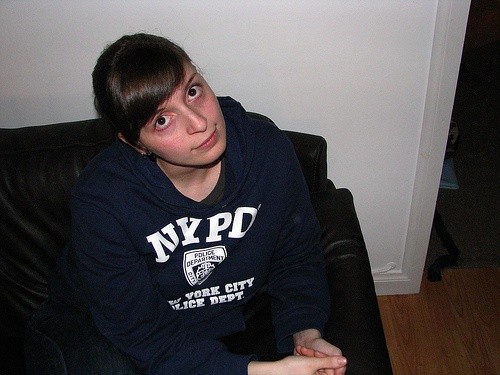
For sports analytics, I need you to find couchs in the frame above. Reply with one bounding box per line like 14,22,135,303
0,116,393,375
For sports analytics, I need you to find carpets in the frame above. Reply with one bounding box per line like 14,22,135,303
427,40,500,268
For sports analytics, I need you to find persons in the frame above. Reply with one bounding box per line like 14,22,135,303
32,32,348,375
